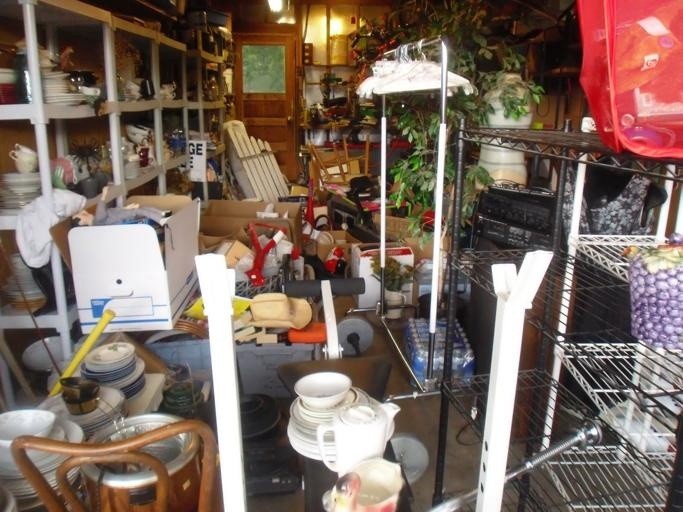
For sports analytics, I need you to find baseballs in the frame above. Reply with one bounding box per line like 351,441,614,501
342,223,348,230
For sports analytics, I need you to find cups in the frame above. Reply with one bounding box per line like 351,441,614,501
323,457,404,512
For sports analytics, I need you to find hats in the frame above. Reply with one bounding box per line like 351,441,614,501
242,293,314,330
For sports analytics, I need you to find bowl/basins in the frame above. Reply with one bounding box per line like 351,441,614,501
293,371,352,408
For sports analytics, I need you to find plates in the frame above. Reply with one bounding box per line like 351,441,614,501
0,331,148,512
0,57,155,312
286,386,396,462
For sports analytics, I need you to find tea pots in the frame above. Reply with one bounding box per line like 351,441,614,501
316,400,402,479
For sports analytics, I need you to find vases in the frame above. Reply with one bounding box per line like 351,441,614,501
474,74,533,192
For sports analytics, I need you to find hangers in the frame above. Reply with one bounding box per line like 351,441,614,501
353,38,470,100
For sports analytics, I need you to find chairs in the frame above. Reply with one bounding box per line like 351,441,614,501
10,418,218,511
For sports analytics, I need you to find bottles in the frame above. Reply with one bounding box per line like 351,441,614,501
401,315,476,388
